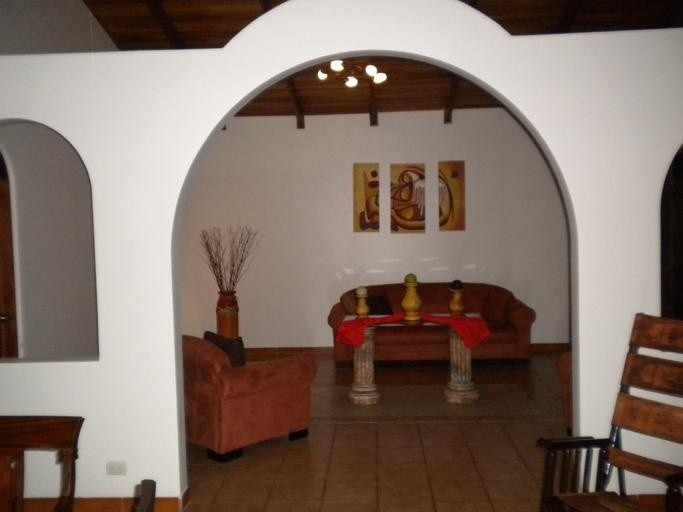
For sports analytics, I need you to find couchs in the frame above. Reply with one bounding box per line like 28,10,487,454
326,281,535,368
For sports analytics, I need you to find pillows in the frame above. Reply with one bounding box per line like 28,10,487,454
355,294,393,315
203,330,245,367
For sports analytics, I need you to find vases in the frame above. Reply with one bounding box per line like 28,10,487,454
215,289,238,339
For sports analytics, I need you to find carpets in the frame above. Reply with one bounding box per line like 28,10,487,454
309,384,560,425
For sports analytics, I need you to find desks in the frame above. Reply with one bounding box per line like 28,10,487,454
338,312,486,406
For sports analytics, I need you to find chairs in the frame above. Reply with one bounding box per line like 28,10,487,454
535,312,682,510
180,332,317,463
